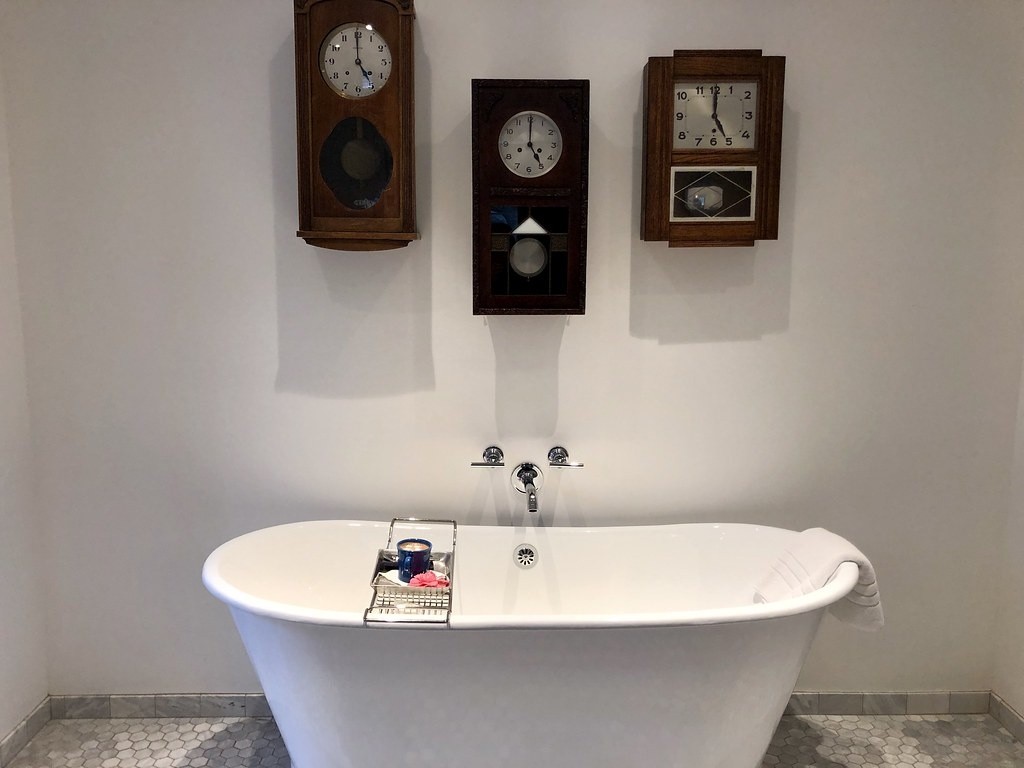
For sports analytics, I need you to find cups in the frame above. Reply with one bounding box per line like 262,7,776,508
397,538,432,583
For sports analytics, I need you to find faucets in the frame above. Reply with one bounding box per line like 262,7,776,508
510,462,544,514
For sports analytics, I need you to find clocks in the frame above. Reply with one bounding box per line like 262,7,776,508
640,49,785,245
293,0,420,252
471,78,586,316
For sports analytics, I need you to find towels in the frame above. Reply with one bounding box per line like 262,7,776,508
750,527,884,634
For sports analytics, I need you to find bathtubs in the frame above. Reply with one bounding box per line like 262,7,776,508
201,520,858,768
